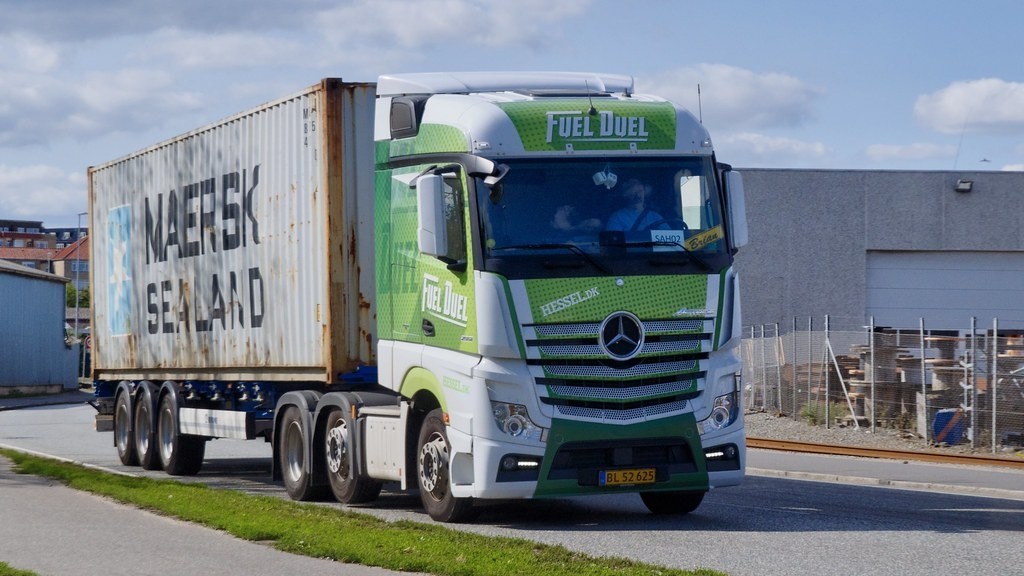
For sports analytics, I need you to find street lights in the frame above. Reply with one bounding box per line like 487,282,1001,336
74,211,88,339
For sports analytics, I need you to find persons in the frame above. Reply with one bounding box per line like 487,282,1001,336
605,178,671,231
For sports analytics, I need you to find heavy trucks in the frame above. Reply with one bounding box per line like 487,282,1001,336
87,71,749,524
